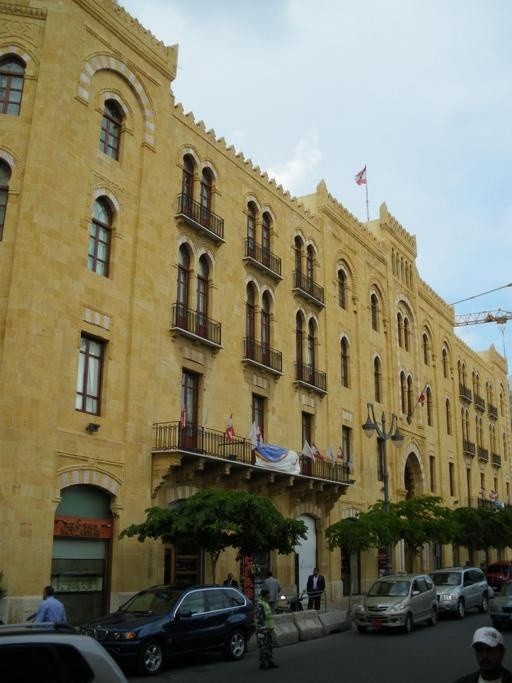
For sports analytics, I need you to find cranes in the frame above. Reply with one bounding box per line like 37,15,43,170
448,282,512,327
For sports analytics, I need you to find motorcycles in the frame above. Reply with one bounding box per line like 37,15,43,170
273,587,307,612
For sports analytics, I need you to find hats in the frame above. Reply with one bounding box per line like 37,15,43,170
469,625,504,650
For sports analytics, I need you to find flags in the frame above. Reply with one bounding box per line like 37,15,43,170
354,165,367,185
224,412,353,474
418,392,425,406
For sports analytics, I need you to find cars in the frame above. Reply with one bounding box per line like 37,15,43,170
354,558,510,634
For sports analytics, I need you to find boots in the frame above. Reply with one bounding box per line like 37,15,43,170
268,660,280,668
259,662,271,670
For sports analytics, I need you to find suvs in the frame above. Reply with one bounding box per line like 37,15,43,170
78,583,256,675
1,620,127,683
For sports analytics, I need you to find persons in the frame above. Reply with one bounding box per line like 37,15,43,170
255,589,279,671
262,571,281,615
31,585,67,630
223,572,239,588
306,567,325,609
454,625,511,682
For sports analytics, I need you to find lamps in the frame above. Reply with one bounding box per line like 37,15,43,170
86,422,100,432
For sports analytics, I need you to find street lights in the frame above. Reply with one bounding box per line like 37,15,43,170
361,401,405,579
